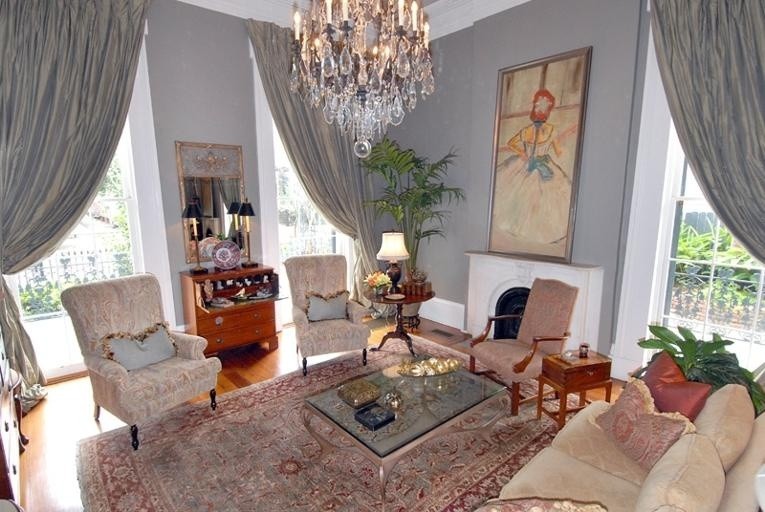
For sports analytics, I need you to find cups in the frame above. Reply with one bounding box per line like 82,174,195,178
579,345,588,358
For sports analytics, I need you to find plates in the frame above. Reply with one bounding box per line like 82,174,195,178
399,357,466,377
560,351,580,364
384,293,406,300
197,238,221,258
211,241,241,270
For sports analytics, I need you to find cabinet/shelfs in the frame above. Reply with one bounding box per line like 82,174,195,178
0,326,28,506
179,264,279,355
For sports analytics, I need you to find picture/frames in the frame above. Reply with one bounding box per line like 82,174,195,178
482,45,596,264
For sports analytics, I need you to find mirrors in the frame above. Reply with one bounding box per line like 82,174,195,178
174,140,249,264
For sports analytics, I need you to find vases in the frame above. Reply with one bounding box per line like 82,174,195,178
373,286,388,296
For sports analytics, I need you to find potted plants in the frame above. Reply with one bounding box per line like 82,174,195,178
355,133,467,317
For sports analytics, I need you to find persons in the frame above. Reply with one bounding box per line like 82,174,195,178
493,90,577,245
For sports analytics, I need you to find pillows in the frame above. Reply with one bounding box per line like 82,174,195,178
474,493,609,512
303,289,352,322
643,351,712,423
99,321,178,372
590,378,695,476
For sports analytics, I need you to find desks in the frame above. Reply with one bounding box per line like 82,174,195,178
535,347,616,433
362,287,436,359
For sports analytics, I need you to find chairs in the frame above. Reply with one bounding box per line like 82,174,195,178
59,269,223,451
467,278,580,417
282,253,373,377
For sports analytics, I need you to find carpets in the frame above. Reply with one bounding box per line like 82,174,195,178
76,330,586,512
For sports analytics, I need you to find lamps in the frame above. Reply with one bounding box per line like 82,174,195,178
286,0,437,159
180,202,210,274
376,230,409,297
226,202,241,246
237,202,259,268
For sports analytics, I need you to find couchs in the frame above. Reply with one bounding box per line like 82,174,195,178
475,375,765,512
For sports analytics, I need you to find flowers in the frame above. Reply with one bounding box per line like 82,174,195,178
364,271,390,287
